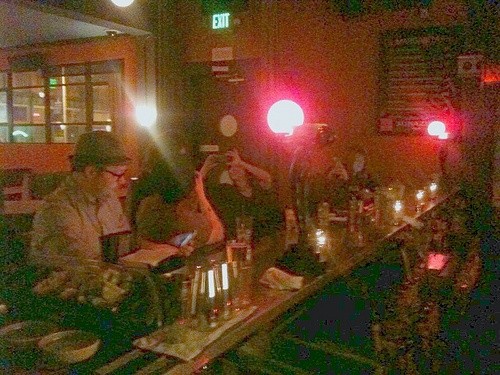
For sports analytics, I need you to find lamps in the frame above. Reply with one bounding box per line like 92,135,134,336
264,98,305,146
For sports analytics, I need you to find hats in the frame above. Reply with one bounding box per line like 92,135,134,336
66,131,132,167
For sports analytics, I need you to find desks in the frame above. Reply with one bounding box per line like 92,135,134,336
0,171,466,375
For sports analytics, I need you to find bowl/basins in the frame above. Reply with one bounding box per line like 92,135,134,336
38,329,100,362
5,320,59,349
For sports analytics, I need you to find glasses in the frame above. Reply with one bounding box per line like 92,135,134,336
94,169,129,179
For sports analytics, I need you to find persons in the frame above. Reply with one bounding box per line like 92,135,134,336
122,127,383,250
26,130,196,264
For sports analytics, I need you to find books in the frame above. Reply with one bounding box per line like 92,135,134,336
116,227,197,269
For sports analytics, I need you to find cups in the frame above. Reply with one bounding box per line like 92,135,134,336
312,203,333,267
344,170,441,250
179,213,255,332
